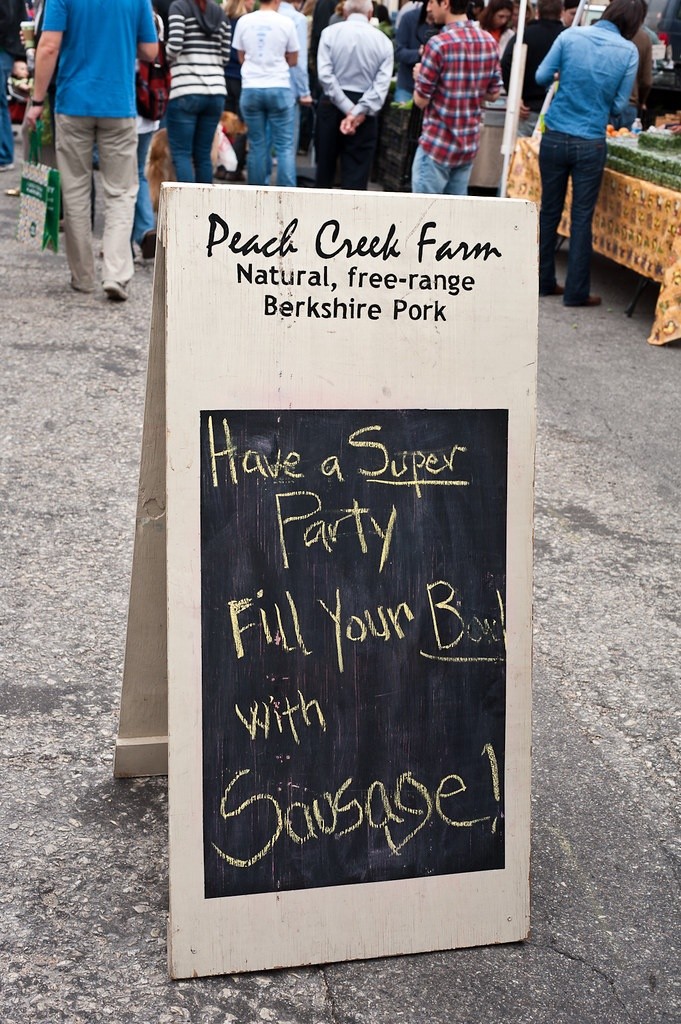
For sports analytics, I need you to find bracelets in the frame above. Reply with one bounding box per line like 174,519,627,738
31,99,44,106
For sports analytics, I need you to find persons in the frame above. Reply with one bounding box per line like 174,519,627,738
608,0,681,129
307,0,535,196
131,0,314,266
10,0,103,230
559,0,581,27
0,0,24,171
534,0,650,310
501,0,569,139
21,0,160,298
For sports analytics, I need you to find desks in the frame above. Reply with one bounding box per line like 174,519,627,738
506,136,680,343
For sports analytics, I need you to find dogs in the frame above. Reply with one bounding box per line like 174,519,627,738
145,111,249,212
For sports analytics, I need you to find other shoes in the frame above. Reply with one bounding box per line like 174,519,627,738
141,230,158,259
102,280,129,300
72,277,95,293
539,284,566,296
565,295,602,307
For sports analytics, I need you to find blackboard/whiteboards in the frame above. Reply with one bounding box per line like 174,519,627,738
114,179,538,979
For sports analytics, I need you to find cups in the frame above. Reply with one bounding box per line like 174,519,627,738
20,21,35,49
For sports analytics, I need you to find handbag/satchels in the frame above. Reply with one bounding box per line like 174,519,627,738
211,124,238,172
18,120,60,253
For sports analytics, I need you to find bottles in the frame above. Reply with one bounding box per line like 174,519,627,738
631,118,643,136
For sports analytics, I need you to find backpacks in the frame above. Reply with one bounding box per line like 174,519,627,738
136,16,173,120
